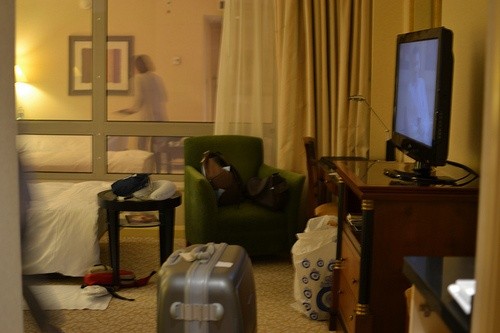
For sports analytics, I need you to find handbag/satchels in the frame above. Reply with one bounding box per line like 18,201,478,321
290,214,337,320
81,264,157,300
248,173,289,208
200,150,241,205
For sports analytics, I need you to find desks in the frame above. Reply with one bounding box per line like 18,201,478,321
402,256,478,333
98,189,182,292
156,137,187,174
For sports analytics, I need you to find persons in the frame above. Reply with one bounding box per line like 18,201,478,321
123,55,172,173
405,46,430,145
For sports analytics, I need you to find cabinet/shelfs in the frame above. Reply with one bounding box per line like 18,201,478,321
321,157,479,333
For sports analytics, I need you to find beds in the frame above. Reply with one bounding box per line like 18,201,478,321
21,150,153,174
21,182,118,278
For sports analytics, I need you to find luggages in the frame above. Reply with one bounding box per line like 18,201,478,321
157,241,257,333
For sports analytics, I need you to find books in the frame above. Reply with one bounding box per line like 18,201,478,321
347,212,363,231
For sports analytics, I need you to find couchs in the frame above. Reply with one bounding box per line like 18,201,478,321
183,135,306,262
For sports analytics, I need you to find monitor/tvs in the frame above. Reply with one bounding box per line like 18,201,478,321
384,27,456,186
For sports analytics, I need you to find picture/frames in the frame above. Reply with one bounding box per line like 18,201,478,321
69,35,135,96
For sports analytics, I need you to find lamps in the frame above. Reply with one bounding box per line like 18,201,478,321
346,95,392,135
